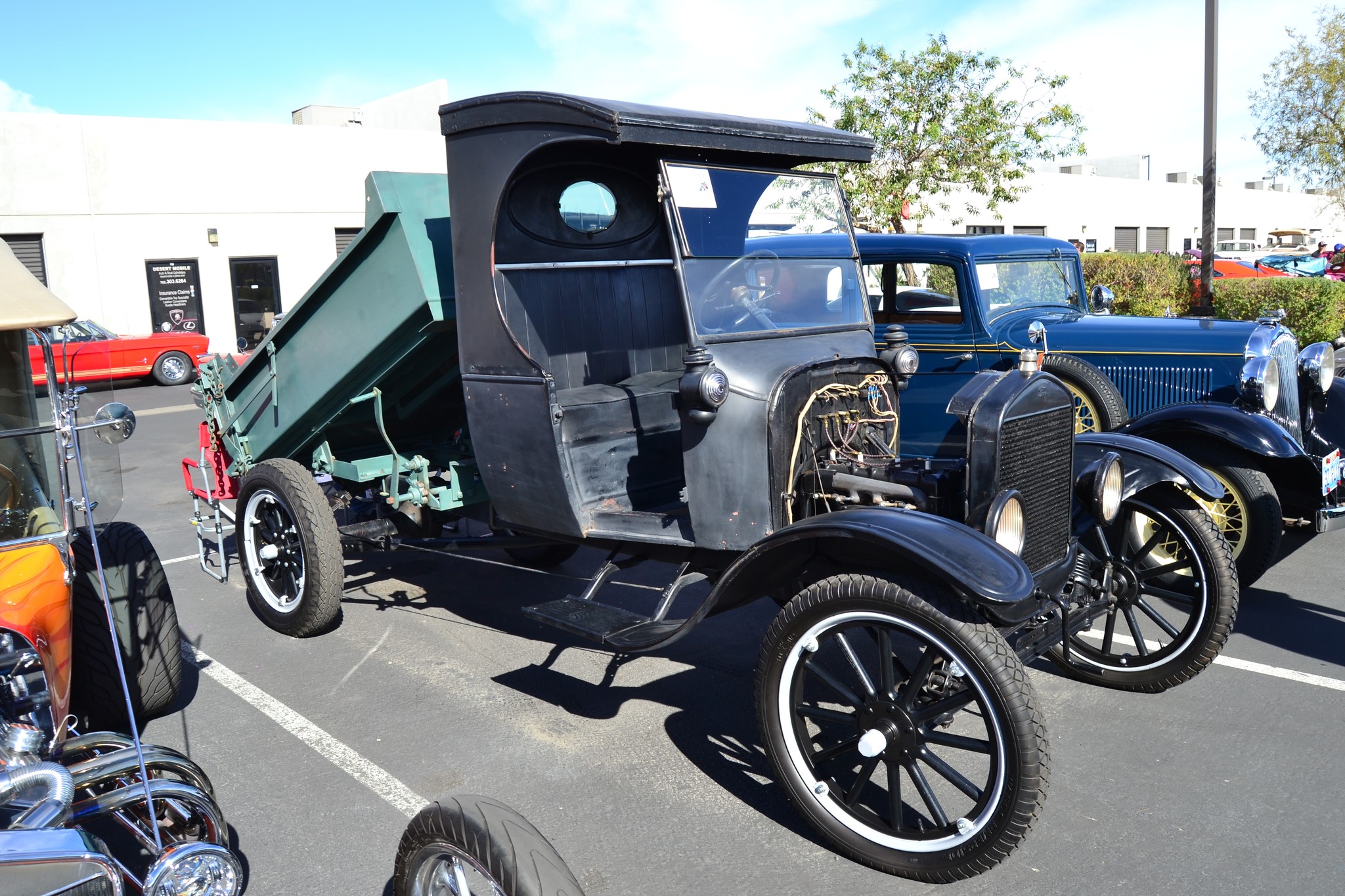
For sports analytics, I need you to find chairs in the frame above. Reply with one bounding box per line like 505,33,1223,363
0,424,67,543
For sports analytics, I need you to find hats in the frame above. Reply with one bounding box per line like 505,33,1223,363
1318,241,1328,247
1334,243,1343,254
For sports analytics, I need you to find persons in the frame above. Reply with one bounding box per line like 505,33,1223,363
1332,242,1345,271
1072,242,1085,254
1257,245,1262,251
1311,241,1328,258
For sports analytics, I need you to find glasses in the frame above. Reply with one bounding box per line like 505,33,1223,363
1321,245,1326,247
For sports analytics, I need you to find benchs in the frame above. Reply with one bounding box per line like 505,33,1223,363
490,262,689,447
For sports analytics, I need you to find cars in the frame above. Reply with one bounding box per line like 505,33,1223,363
2,89,1345,895
